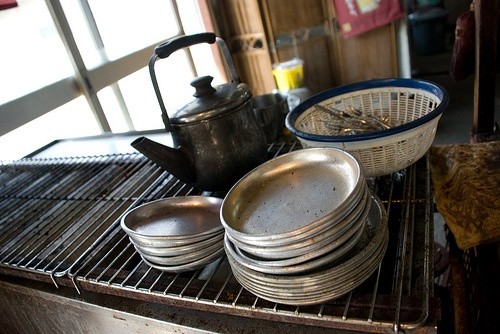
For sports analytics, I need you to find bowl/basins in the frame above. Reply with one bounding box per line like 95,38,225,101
251,93,289,142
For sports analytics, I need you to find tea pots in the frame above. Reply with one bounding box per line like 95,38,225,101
129,32,270,191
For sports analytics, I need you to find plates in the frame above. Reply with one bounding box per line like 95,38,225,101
120,147,390,307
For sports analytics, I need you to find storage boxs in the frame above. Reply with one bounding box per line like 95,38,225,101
273,58,305,90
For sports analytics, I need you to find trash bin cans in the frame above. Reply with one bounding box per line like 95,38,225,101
408,7,449,55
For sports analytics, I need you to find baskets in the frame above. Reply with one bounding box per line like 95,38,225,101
284,77,449,175
